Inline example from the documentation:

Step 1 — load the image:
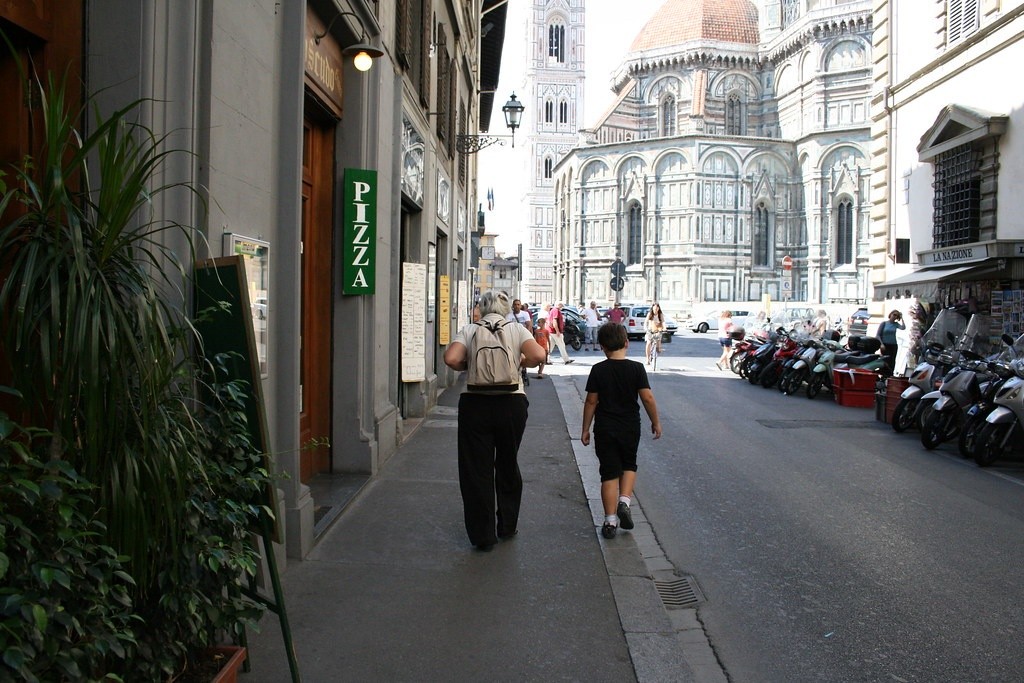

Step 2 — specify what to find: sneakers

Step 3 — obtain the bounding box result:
[602,521,618,539]
[616,502,634,530]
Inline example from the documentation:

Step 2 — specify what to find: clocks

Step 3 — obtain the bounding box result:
[481,246,495,260]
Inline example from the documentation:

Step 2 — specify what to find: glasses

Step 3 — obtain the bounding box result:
[892,313,899,318]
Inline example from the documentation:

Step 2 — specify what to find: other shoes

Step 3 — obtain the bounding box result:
[545,362,553,365]
[716,361,723,371]
[593,348,599,351]
[477,545,493,551]
[499,534,516,541]
[565,360,574,364]
[538,370,543,378]
[585,348,589,351]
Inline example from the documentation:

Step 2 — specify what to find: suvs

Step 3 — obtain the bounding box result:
[849,306,868,336]
[602,306,650,341]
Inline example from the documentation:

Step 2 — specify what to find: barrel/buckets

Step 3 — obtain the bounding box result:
[885,373,912,424]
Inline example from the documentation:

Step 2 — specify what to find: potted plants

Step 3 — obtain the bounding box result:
[0,27,333,683]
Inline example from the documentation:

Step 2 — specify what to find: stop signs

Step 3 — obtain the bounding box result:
[782,257,792,270]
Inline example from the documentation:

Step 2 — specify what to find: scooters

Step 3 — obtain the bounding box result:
[891,330,1024,469]
[727,316,890,399]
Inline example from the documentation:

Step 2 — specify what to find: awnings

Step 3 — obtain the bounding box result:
[874,260,999,302]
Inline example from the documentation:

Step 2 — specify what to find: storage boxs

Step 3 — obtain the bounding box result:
[875,393,887,423]
[831,386,874,408]
[833,368,878,391]
[886,377,911,424]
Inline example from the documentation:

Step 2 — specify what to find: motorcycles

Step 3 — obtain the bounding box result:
[561,312,582,351]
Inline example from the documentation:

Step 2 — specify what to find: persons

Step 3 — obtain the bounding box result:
[581,322,661,539]
[604,302,626,324]
[506,299,551,378]
[642,303,667,365]
[875,310,906,382]
[546,300,575,365]
[473,301,481,323]
[443,290,547,553]
[578,300,601,352]
[716,310,735,371]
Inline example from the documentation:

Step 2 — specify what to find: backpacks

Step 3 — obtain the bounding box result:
[467,320,521,392]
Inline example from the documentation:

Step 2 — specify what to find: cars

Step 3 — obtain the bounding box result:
[685,311,757,333]
[664,317,677,336]
[527,305,609,332]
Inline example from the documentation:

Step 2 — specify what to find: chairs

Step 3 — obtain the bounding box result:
[637,312,644,317]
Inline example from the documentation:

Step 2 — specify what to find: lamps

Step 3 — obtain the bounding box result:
[457,91,525,155]
[314,12,384,72]
